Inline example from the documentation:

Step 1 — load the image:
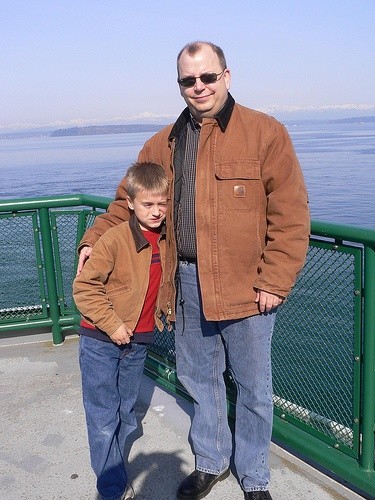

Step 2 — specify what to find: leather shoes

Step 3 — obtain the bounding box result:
[244,492,272,500]
[177,465,230,500]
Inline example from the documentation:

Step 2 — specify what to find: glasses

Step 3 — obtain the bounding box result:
[177,71,225,87]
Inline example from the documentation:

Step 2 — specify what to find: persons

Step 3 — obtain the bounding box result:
[72,39,311,499]
[71,160,180,500]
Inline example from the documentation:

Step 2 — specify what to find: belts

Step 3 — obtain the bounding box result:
[178,255,197,265]
